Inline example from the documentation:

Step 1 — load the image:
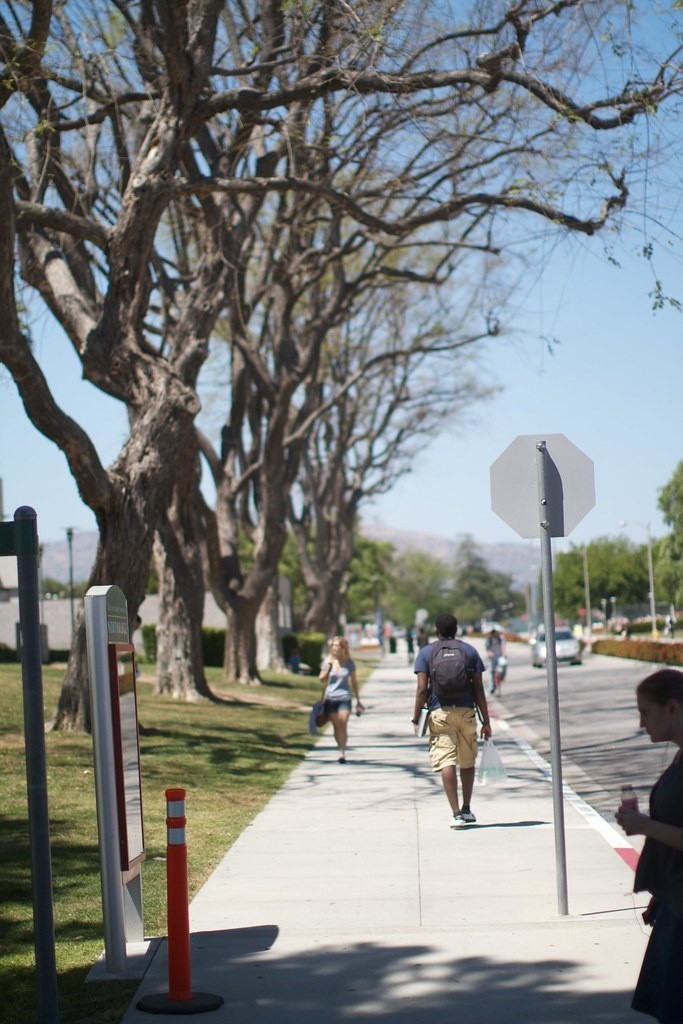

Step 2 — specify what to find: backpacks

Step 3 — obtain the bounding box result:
[431,640,469,699]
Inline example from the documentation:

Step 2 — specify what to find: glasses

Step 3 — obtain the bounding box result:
[331,643,342,647]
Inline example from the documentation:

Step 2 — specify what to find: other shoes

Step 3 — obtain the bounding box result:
[339,757,345,763]
[461,812,476,824]
[451,816,468,830]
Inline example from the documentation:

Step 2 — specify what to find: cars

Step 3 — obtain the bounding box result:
[530,624,581,669]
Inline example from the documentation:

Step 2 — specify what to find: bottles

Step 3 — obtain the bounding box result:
[621,783,641,835]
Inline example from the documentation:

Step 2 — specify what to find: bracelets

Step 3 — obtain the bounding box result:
[411,719,418,725]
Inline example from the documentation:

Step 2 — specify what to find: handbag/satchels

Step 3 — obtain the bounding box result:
[478,736,506,784]
[317,704,328,726]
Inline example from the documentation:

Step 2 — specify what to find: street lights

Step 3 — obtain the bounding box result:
[618,518,657,638]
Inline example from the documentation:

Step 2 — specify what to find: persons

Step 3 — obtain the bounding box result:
[417,627,428,652]
[615,669,683,1024]
[414,613,492,829]
[405,628,415,663]
[486,629,506,694]
[319,636,366,764]
[665,612,677,640]
